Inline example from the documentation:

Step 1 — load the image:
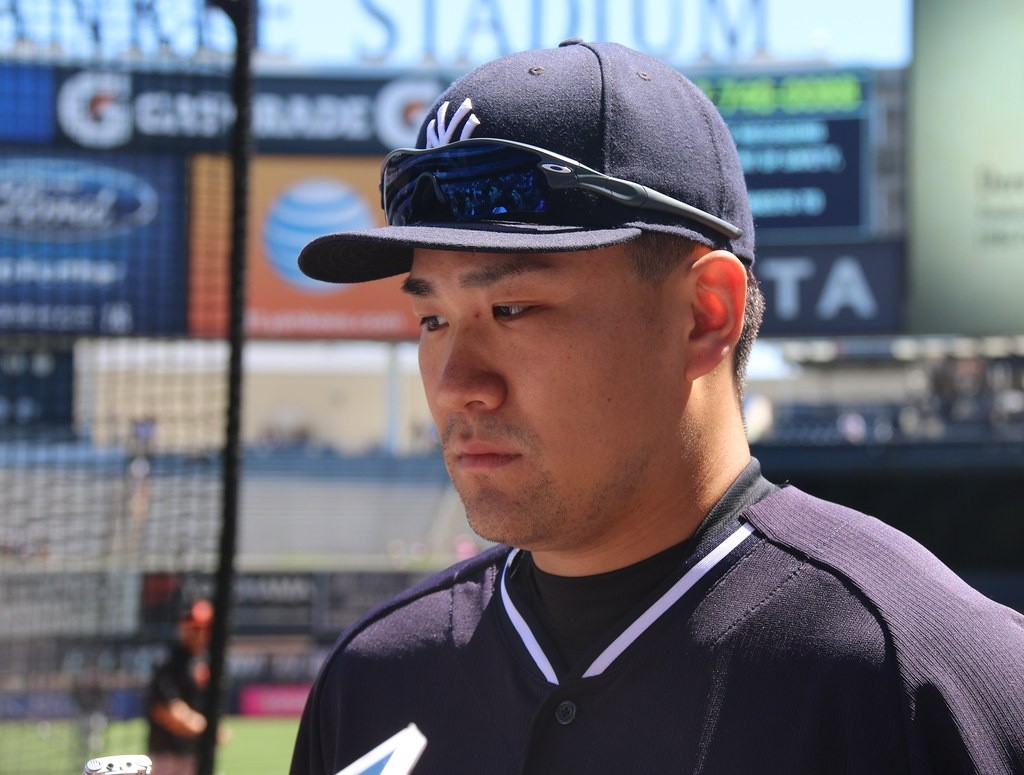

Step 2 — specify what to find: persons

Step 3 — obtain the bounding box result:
[289,36,1023,775]
[142,601,226,774]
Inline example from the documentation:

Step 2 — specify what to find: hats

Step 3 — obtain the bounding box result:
[297,39,756,284]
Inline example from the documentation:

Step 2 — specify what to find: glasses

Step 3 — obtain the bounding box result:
[380,137,744,239]
